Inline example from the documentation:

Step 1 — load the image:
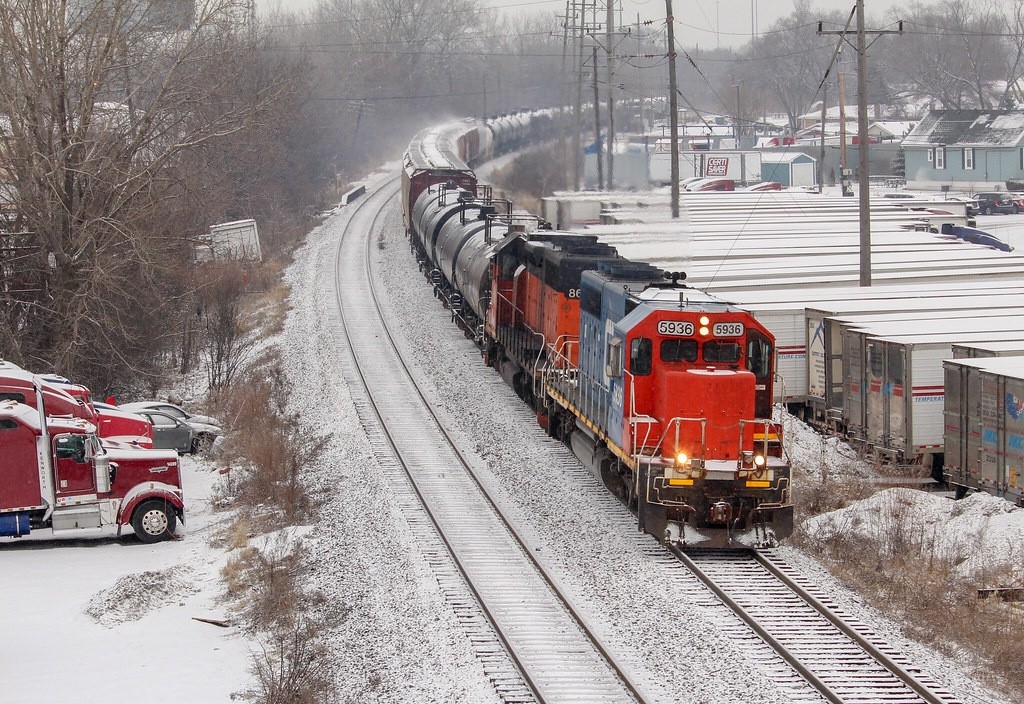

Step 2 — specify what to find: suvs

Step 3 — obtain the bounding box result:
[1009,192,1024,214]
[972,192,1013,215]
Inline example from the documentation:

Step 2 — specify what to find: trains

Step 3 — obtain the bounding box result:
[399,91,797,553]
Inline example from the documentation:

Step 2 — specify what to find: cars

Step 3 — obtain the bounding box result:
[946,197,980,218]
[125,409,223,455]
[754,122,783,133]
[118,401,221,428]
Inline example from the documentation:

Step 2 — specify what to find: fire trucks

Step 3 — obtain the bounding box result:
[0,358,154,450]
[0,398,185,546]
